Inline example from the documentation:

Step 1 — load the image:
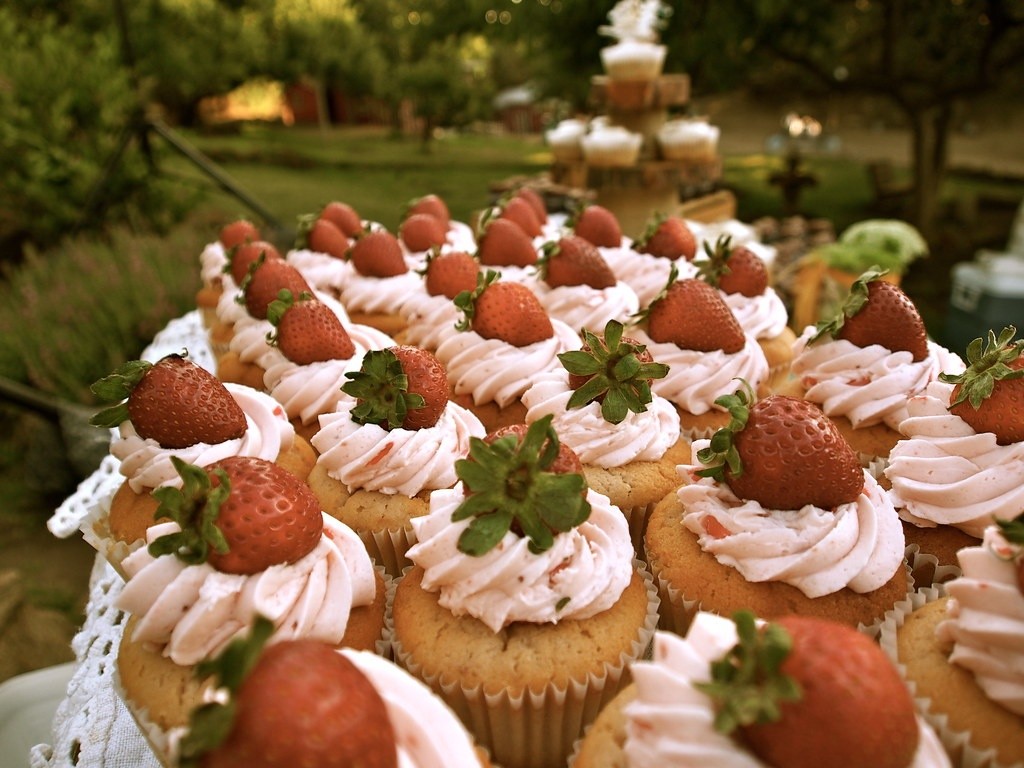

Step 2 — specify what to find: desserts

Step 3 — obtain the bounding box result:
[77,36,1024,768]
